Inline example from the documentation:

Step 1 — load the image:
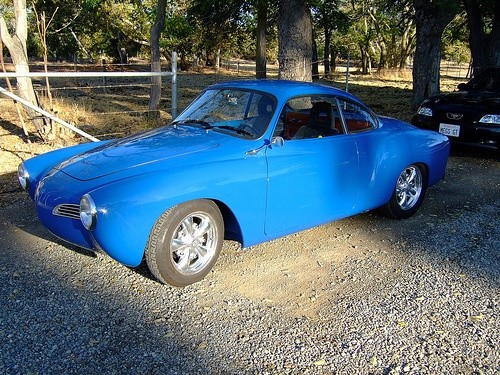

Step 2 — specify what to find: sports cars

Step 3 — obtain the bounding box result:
[17,74,452,288]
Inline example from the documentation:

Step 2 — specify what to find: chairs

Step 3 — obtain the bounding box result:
[237,95,284,139]
[290,101,339,140]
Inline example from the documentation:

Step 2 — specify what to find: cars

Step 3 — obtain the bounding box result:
[410,66,500,162]
